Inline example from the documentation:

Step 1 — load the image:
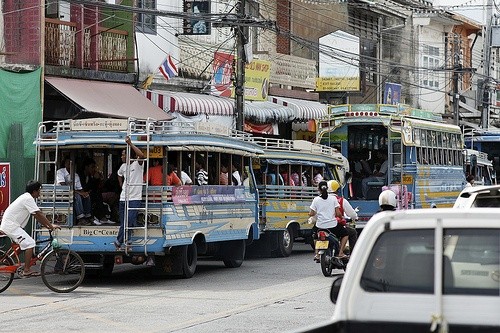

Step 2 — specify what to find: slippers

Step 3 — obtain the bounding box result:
[314,255,320,259]
[23,272,41,276]
[337,255,347,258]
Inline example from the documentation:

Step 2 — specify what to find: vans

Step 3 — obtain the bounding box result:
[441,184,500,288]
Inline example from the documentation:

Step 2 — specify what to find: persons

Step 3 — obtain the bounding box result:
[466,175,474,187]
[267,166,323,199]
[143,154,250,186]
[308,180,358,260]
[0,180,61,277]
[350,149,388,199]
[76,158,116,225]
[115,136,144,249]
[55,157,102,226]
[379,190,397,211]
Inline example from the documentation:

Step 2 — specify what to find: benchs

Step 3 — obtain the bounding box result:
[368,171,388,185]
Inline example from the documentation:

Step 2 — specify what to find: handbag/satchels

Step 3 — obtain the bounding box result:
[308,216,316,225]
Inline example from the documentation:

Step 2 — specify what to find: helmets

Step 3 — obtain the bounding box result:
[327,179,340,193]
[379,189,397,207]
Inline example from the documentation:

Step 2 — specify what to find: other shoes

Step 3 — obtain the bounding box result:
[77,218,96,226]
[100,219,117,224]
[128,243,132,245]
[93,219,102,226]
[115,241,121,249]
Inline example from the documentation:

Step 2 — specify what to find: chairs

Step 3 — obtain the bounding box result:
[403,253,454,289]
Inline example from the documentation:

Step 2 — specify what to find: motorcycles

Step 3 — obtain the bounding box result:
[314,206,360,277]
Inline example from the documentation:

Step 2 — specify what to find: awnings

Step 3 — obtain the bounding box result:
[267,96,328,121]
[43,76,176,132]
[138,88,236,115]
[459,120,498,129]
[220,96,294,122]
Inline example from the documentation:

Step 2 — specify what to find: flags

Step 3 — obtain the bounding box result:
[158,55,178,81]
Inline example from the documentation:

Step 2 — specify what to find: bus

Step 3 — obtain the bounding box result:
[30,116,266,279]
[232,137,353,257]
[314,102,498,232]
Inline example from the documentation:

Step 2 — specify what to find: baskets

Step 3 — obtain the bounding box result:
[53,230,74,244]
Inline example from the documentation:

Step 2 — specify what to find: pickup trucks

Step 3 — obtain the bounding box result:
[293,206,500,333]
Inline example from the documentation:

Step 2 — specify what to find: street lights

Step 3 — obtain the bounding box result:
[377,24,407,104]
[451,78,484,125]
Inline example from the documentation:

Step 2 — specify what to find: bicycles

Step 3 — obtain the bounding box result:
[0,226,86,294]
